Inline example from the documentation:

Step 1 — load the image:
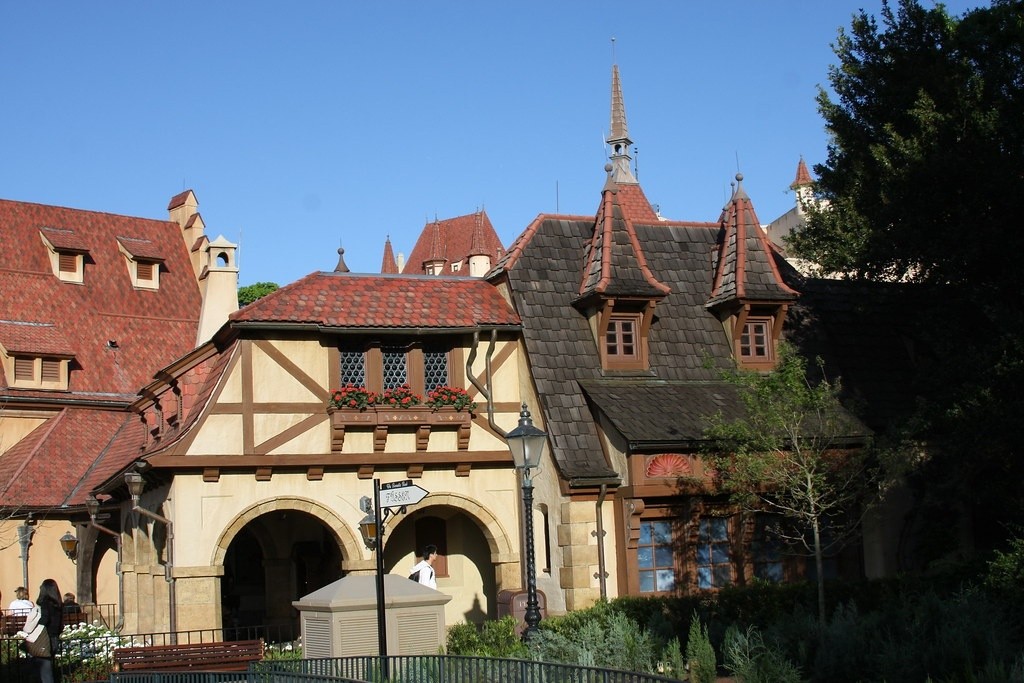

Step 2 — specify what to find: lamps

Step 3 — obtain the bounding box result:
[85,494,99,519]
[124,473,148,507]
[59,529,79,565]
[358,497,386,550]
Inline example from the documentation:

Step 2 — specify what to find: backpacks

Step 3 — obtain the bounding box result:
[408,566,433,583]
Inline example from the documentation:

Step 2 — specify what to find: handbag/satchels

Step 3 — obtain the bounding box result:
[19,625,53,658]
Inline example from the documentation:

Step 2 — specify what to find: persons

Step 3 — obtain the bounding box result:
[22,579,64,683]
[9,586,35,616]
[61,592,81,613]
[407,545,437,591]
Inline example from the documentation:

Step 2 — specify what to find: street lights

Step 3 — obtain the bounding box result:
[503,403,550,650]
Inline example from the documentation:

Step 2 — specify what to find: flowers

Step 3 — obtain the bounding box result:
[327,383,478,411]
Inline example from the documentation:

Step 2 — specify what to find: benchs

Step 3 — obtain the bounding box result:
[116,639,265,672]
[1,613,89,634]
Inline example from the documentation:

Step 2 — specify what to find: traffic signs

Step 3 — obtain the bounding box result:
[379,480,430,508]
